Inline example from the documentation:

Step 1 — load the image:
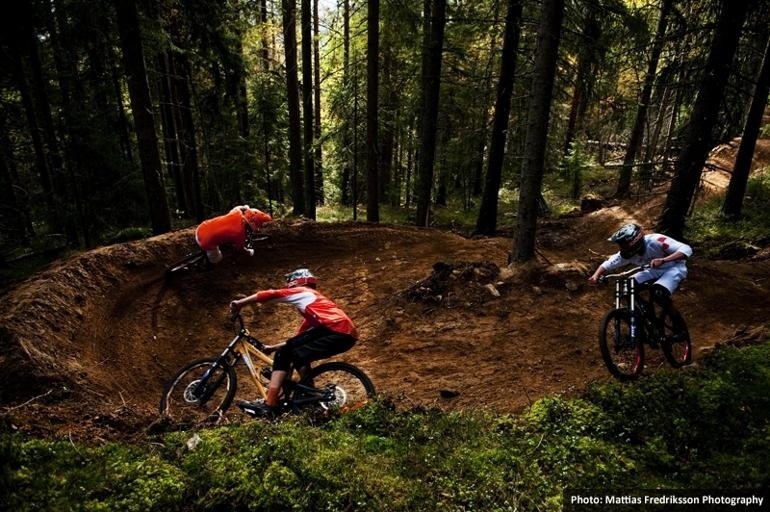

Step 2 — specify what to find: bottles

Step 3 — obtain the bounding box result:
[259,367,273,380]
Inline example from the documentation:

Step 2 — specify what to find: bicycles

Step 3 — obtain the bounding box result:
[164,227,275,277]
[587,257,696,380]
[158,302,379,429]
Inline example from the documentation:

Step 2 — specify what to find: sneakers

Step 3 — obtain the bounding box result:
[672,331,688,342]
[239,399,277,420]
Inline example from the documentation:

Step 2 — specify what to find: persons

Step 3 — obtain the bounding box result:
[195,204,272,264]
[587,223,693,349]
[229,268,358,421]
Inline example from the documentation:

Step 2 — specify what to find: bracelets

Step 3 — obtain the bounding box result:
[660,258,666,264]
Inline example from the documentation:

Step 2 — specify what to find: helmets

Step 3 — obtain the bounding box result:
[245,208,271,230]
[612,224,645,259]
[284,268,319,288]
